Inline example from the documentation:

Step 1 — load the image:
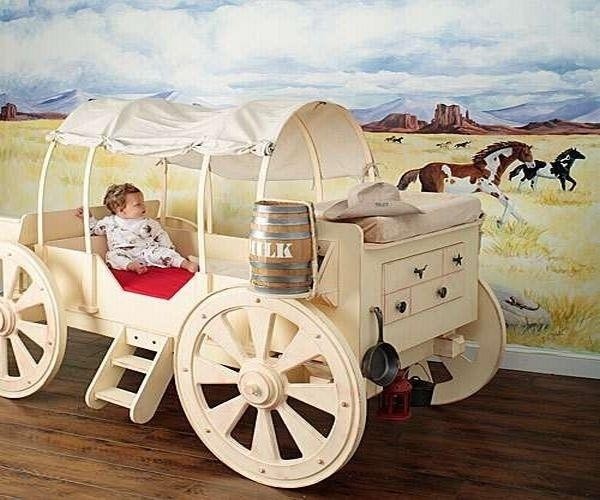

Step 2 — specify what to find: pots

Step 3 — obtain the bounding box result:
[362,306,400,386]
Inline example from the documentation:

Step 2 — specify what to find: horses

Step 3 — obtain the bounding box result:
[383,135,395,143]
[507,146,588,195]
[436,141,451,150]
[454,141,469,149]
[392,136,404,144]
[396,141,537,228]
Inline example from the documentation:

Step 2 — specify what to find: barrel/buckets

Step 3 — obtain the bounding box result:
[403,362,434,408]
[249,200,316,294]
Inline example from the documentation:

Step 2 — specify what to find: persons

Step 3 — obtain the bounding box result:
[74,184,201,278]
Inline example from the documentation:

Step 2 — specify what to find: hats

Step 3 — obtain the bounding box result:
[322,178,425,222]
